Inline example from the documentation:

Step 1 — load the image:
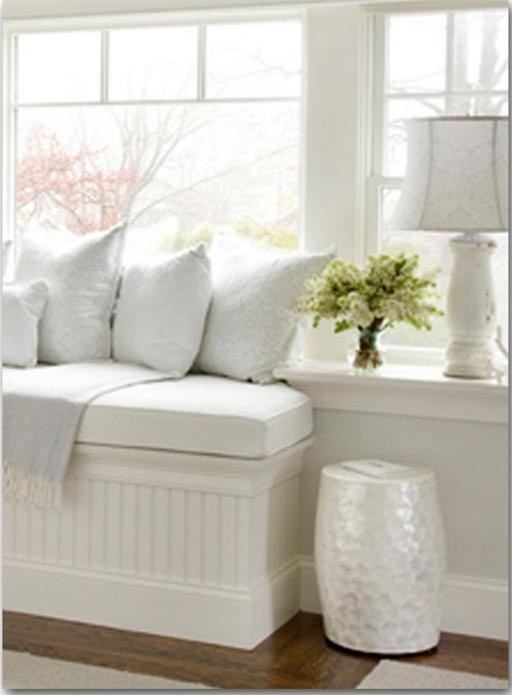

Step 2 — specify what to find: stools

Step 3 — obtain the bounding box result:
[314,459,446,655]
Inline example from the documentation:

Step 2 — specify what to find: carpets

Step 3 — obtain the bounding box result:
[2,650,222,689]
[354,658,508,689]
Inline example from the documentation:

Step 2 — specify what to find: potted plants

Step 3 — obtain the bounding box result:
[293,249,447,370]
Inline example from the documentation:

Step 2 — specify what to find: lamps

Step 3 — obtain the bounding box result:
[390,115,508,378]
